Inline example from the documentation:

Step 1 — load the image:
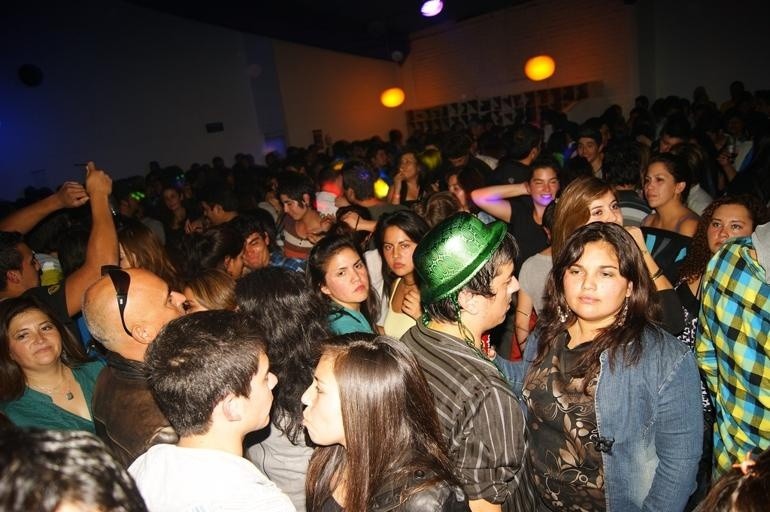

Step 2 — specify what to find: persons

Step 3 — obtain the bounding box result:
[384,111,575,155]
[400,210,532,512]
[603,148,652,227]
[127,310,295,511]
[428,135,494,192]
[230,214,310,272]
[418,148,443,193]
[115,151,269,189]
[576,127,618,181]
[335,159,410,234]
[444,164,483,213]
[333,204,378,253]
[693,444,770,511]
[274,173,331,262]
[693,218,769,480]
[187,185,247,259]
[514,191,563,357]
[119,182,165,244]
[266,136,390,194]
[577,81,770,189]
[378,210,434,344]
[236,268,334,511]
[0,300,108,435]
[313,165,345,217]
[115,223,186,291]
[1,161,118,354]
[305,235,379,336]
[670,142,712,215]
[82,267,187,469]
[296,330,472,512]
[366,144,388,169]
[631,158,701,288]
[674,197,760,506]
[0,421,146,511]
[493,123,546,186]
[184,268,237,313]
[551,176,685,342]
[480,221,704,512]
[387,150,431,213]
[155,184,189,231]
[470,166,561,258]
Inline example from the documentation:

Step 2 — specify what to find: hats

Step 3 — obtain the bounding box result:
[414,212,508,304]
[575,129,600,141]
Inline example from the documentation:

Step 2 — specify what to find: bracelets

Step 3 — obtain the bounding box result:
[649,267,665,282]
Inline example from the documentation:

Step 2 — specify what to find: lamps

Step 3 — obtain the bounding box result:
[417,0,445,19]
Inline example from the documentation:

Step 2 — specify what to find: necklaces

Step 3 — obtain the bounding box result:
[26,370,74,402]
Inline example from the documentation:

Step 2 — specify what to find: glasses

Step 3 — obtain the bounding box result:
[101,265,134,336]
